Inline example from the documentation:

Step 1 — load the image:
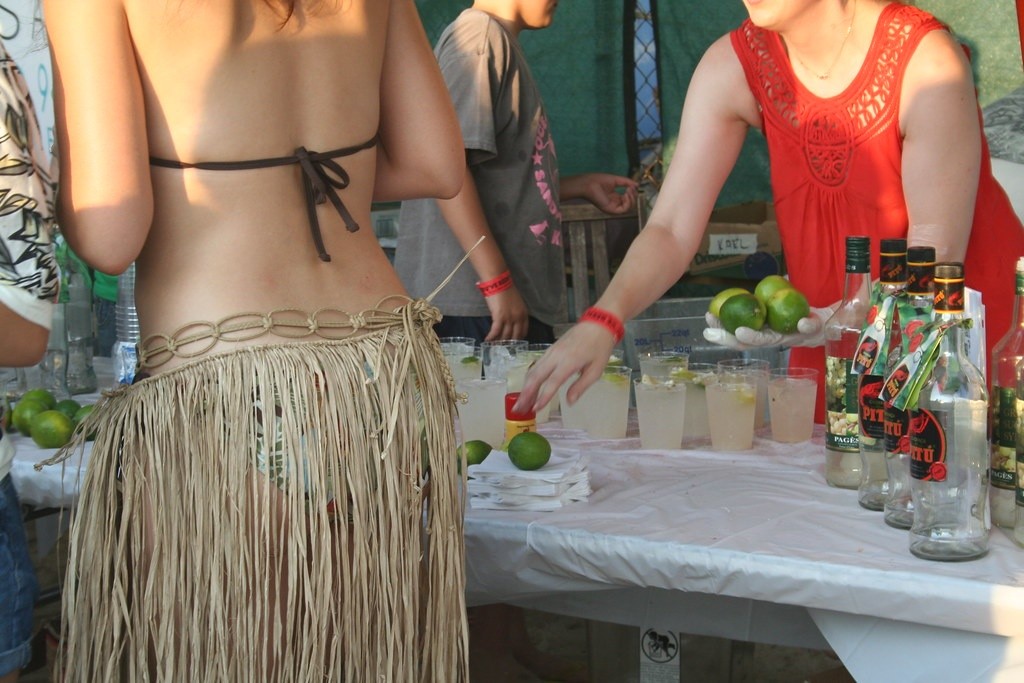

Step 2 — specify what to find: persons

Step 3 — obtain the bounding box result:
[0,0,466,683]
[512,0,1024,424]
[394,0,641,379]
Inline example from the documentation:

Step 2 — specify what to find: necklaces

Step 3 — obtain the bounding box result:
[795,0,857,79]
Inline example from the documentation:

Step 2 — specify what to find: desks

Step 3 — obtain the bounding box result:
[0,355,1024,683]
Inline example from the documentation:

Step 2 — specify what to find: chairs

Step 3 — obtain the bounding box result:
[552,193,657,339]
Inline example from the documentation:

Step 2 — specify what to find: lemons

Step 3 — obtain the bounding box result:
[5,386,100,449]
[507,432,552,470]
[456,440,494,475]
[708,275,811,336]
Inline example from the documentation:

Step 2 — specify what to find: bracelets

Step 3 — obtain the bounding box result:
[475,271,512,297]
[577,307,625,342]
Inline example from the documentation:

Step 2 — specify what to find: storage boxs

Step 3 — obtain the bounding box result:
[686,200,782,277]
[621,297,739,372]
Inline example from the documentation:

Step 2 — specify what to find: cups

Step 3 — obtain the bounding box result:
[441,336,817,449]
[114,255,140,390]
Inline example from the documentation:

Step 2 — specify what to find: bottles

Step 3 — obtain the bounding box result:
[0,225,98,395]
[824,236,1024,563]
